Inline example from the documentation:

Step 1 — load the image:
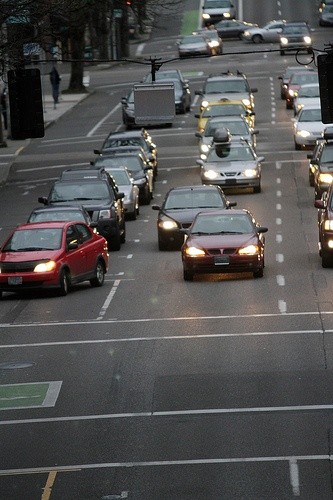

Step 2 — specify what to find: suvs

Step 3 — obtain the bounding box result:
[38,166,125,251]
[194,69,258,111]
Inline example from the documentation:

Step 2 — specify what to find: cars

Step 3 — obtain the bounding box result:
[0,220,110,296]
[178,208,268,282]
[139,70,183,83]
[121,90,135,125]
[102,127,158,156]
[195,115,260,156]
[278,66,333,269]
[194,100,255,134]
[175,0,333,60]
[196,140,265,193]
[90,154,153,204]
[156,79,192,113]
[93,146,151,166]
[151,184,238,252]
[26,205,97,233]
[103,165,140,221]
